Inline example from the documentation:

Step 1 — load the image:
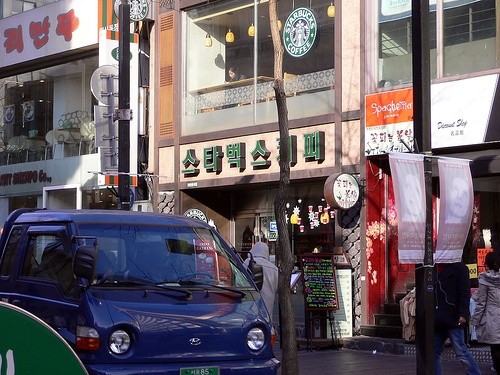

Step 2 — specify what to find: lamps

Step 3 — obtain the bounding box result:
[327,0,335,18]
[204,18,212,47]
[276,0,282,31]
[225,11,235,42]
[248,6,255,36]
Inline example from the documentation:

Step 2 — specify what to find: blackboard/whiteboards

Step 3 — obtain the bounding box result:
[299,253,340,310]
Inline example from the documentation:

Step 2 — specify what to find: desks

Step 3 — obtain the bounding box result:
[196,74,274,113]
[58,127,86,155]
[24,135,45,159]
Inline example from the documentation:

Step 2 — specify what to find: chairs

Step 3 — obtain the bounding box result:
[0,121,96,165]
[33,241,73,282]
[268,72,300,101]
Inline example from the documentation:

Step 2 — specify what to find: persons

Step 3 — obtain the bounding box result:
[471,251,500,375]
[431,262,481,375]
[241,241,279,324]
[222,66,247,110]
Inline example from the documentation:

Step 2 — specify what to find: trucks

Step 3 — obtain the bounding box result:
[0,206,281,375]
